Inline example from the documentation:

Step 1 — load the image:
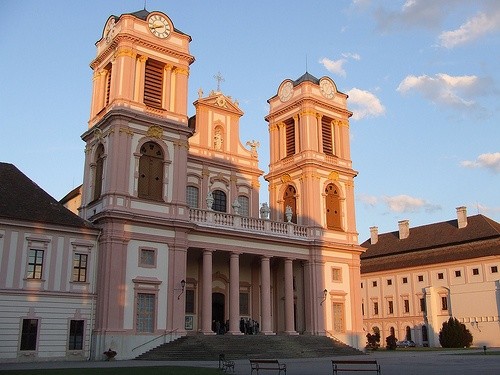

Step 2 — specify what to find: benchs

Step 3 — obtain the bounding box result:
[332,360,381,375]
[250,359,288,375]
[219,353,236,372]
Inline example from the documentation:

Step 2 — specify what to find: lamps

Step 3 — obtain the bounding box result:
[178,280,185,300]
[321,289,328,304]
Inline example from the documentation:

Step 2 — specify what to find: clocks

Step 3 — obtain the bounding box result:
[103,15,117,44]
[146,11,175,39]
[319,76,338,99]
[277,79,294,102]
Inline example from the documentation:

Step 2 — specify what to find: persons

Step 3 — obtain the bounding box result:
[239,317,259,336]
[212,319,229,335]
